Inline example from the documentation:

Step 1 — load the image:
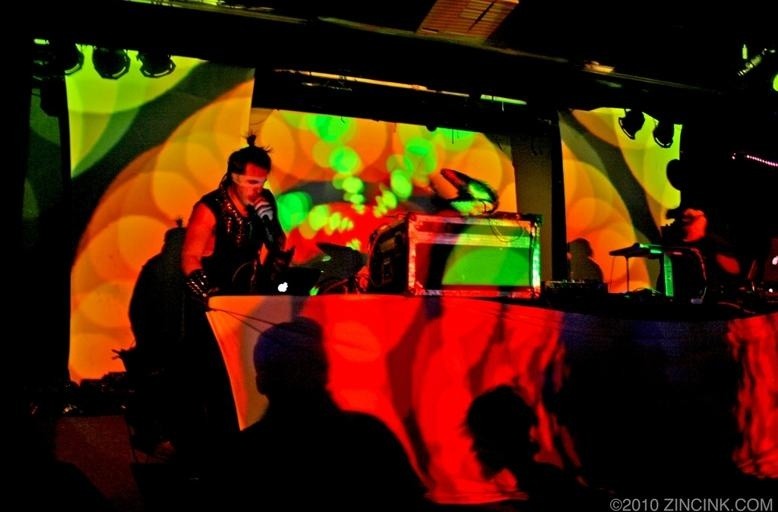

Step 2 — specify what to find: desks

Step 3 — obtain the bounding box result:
[206,291,774,505]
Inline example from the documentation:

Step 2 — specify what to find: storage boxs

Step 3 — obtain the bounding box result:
[370,215,543,298]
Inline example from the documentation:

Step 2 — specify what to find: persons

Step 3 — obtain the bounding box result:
[181,132,285,299]
[656,204,711,305]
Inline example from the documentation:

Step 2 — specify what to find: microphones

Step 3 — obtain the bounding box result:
[251,194,276,243]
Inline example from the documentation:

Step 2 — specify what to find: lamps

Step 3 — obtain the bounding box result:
[43,41,84,77]
[92,50,131,81]
[137,52,177,80]
[620,108,646,141]
[652,120,675,148]
